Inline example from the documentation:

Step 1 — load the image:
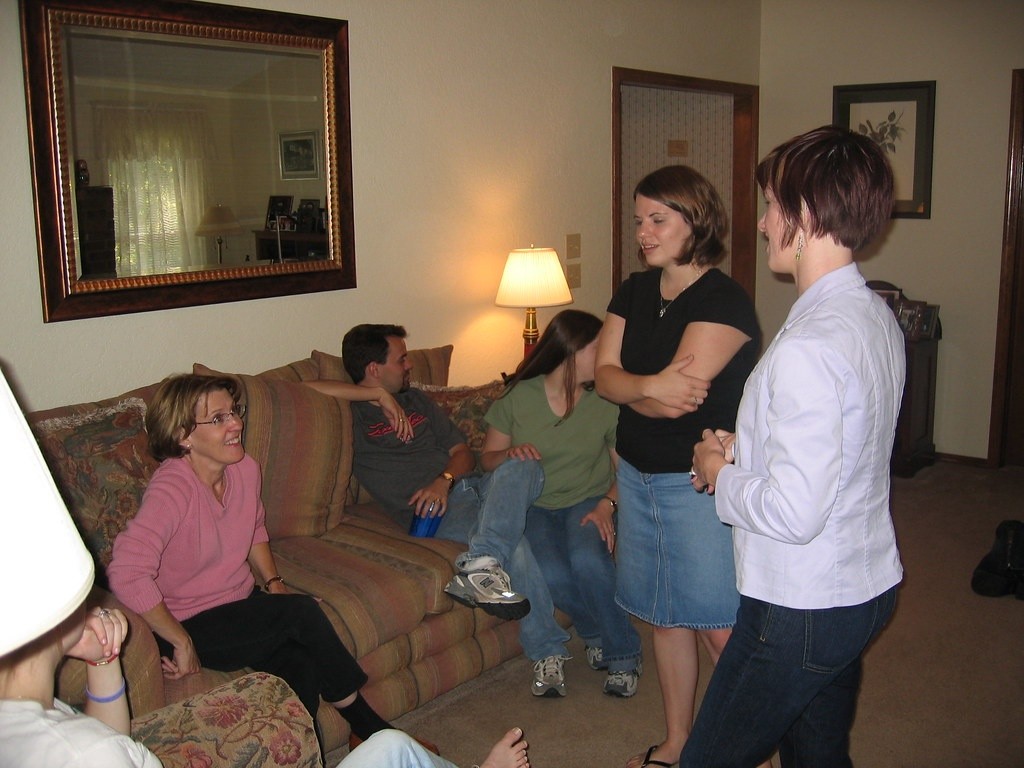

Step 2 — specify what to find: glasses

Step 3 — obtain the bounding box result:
[196,404,247,427]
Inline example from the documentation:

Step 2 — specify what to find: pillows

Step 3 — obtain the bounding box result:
[194,360,345,540]
[409,376,507,469]
[24,397,168,586]
[251,356,319,382]
[311,345,455,387]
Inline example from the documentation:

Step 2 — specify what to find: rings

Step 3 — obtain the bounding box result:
[98,609,111,616]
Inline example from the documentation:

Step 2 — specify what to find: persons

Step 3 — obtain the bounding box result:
[673,122,911,768]
[592,162,766,767]
[0,586,533,768]
[301,324,574,699]
[482,307,646,698]
[106,372,441,765]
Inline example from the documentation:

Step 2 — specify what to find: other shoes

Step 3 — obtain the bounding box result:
[348,732,441,757]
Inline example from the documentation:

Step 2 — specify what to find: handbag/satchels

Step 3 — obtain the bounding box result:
[968,520,1023,600]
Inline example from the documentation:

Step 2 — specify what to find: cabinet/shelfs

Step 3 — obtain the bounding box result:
[252,230,329,263]
[75,186,117,279]
[887,336,936,480]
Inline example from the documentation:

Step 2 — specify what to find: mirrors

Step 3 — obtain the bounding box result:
[18,0,357,325]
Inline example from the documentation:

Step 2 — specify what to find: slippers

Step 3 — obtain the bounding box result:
[624,745,680,768]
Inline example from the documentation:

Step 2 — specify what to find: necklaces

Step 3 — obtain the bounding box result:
[657,262,707,318]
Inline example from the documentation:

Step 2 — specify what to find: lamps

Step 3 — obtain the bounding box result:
[195,202,246,266]
[495,243,575,361]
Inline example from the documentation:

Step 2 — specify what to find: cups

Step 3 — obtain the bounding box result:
[408,496,445,538]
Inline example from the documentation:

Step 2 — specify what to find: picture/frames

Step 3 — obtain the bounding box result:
[299,199,320,233]
[278,129,322,182]
[833,80,940,222]
[265,195,294,232]
[919,305,940,339]
[894,298,927,343]
[278,215,297,232]
[869,286,902,311]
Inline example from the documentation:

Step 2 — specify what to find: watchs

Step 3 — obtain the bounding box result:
[604,494,618,513]
[437,473,455,488]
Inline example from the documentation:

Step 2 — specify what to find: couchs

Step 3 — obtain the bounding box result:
[22,345,574,748]
[128,671,327,768]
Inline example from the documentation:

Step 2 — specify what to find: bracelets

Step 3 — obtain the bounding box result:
[85,679,127,705]
[264,575,283,589]
[87,655,120,669]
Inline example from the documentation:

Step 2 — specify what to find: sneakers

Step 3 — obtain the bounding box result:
[585,647,604,670]
[443,568,531,621]
[530,647,568,697]
[603,662,643,697]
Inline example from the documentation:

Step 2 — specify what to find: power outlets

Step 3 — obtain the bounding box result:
[566,262,581,290]
